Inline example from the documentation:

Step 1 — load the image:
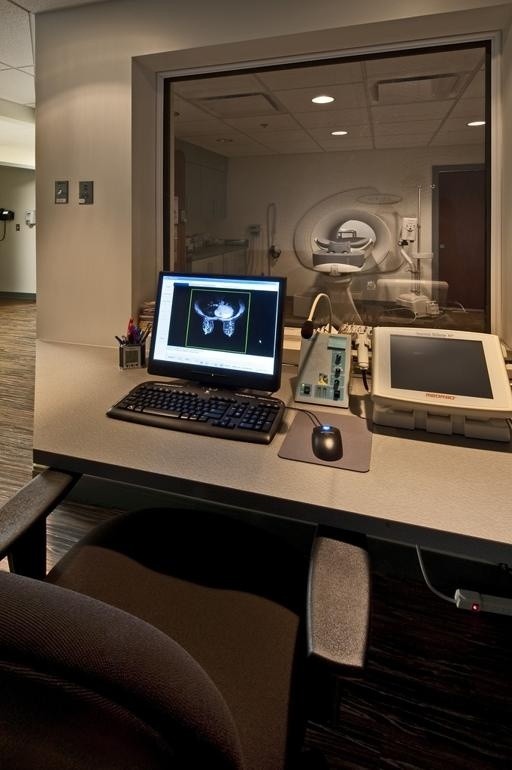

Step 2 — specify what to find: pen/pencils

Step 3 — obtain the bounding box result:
[115,317,152,345]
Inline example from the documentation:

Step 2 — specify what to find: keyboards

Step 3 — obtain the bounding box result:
[106,381,285,444]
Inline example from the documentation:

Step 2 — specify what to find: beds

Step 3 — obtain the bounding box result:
[313,238,372,273]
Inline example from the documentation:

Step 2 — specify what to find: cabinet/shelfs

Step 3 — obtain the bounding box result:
[188,246,248,275]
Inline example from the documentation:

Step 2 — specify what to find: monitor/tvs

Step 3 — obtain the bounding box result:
[370,326,512,419]
[147,271,287,397]
[376,279,449,309]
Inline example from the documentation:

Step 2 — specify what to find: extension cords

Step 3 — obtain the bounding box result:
[454,588,512,616]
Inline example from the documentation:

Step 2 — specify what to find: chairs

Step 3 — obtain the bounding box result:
[0,468,370,770]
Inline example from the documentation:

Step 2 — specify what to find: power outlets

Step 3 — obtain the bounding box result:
[78,181,93,204]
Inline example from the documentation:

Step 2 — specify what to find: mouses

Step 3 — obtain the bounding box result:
[312,426,343,461]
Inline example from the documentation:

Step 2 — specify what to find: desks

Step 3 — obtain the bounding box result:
[32,341,512,566]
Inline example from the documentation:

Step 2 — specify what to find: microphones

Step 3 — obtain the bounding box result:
[294,293,352,410]
